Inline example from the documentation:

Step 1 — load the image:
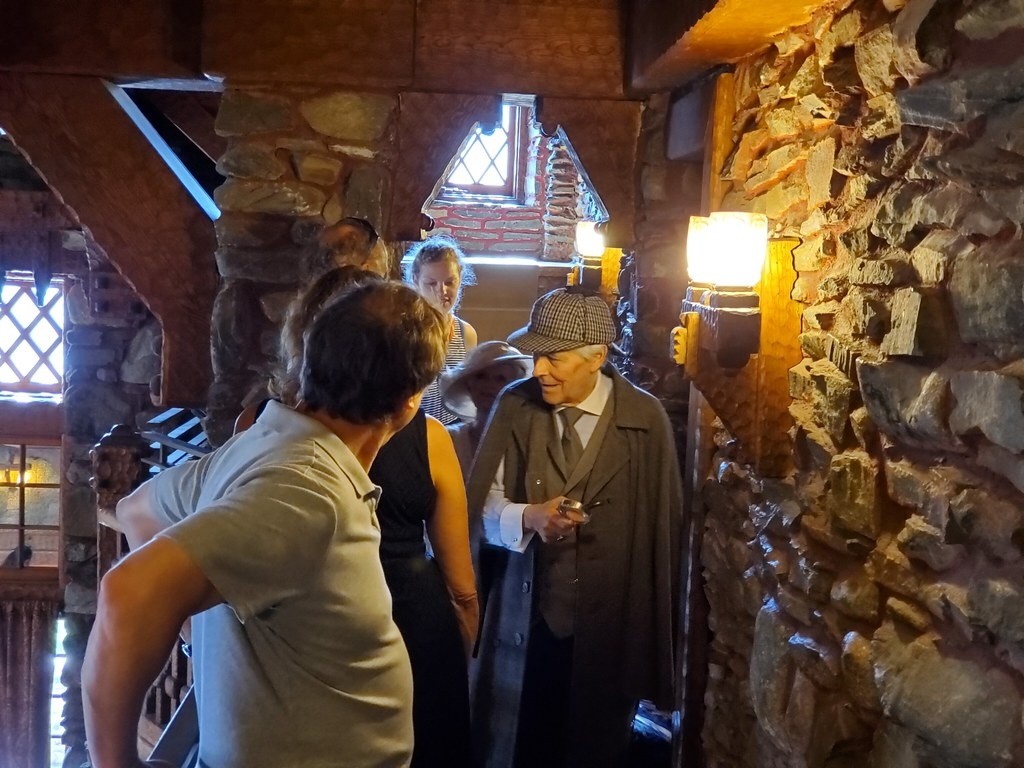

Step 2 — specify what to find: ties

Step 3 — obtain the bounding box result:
[559,407,585,471]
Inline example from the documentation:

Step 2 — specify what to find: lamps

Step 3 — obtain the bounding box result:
[704,212,766,308]
[685,215,709,303]
[574,219,602,288]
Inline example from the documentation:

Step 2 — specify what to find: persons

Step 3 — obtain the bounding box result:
[440,338,536,486]
[402,232,480,427]
[234,265,480,768]
[81,280,453,768]
[456,287,683,768]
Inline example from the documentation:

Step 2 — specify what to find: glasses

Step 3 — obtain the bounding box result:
[348,217,378,265]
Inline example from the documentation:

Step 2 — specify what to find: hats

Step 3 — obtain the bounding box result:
[504,284,617,358]
[443,337,534,419]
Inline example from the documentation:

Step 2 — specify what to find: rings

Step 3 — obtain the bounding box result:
[557,534,565,542]
